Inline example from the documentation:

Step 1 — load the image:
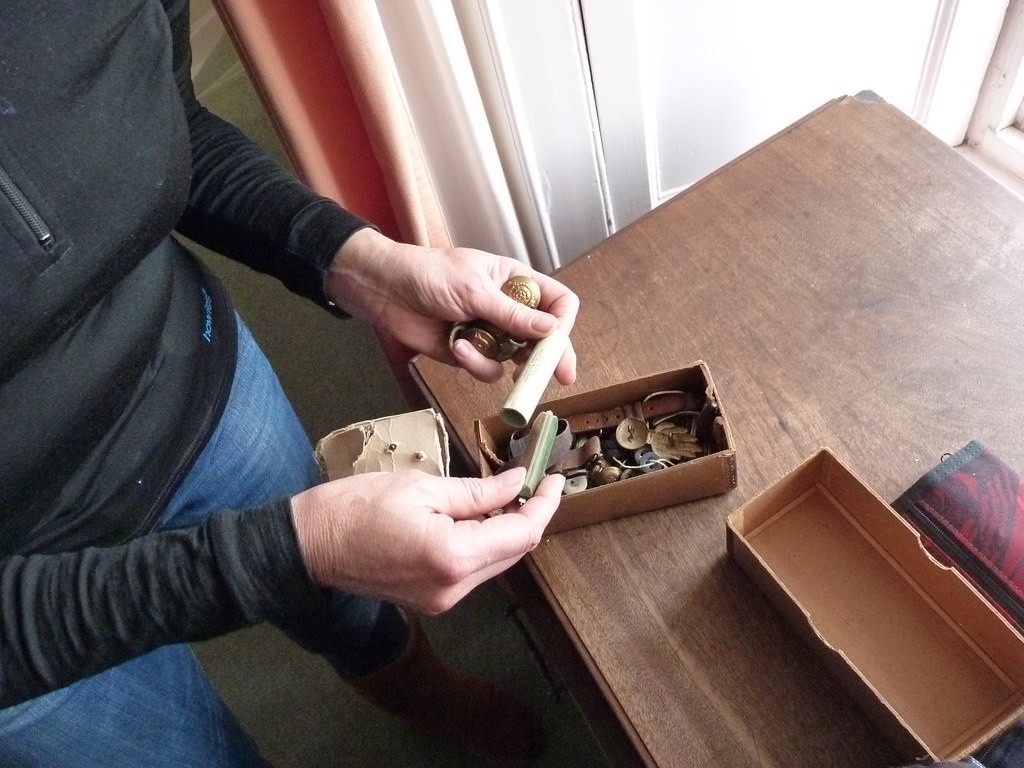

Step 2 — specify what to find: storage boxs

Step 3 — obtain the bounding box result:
[474,359,737,535]
[727,446,1024,765]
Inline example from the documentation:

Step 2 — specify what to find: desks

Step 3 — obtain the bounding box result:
[407,90,1024,768]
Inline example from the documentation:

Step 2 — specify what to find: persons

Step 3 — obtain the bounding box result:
[0,1,584,768]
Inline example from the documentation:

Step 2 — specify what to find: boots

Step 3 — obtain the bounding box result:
[346,604,546,768]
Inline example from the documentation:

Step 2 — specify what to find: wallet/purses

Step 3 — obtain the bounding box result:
[890,438,1024,634]
